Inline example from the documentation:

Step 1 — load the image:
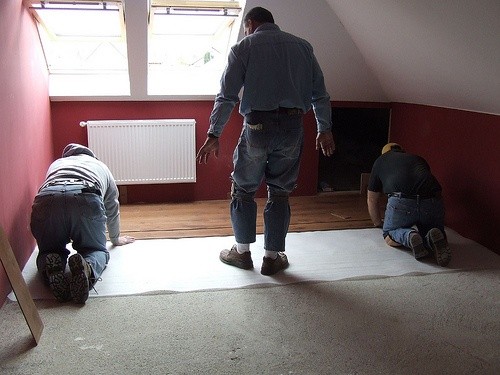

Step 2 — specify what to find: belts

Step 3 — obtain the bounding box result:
[390,193,419,199]
[272,108,303,114]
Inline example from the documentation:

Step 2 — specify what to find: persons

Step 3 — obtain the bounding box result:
[196,6,335,276]
[29,144,135,303]
[367,143,453,266]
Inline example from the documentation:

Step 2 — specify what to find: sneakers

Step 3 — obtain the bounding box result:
[45,252,66,298]
[69,253,91,305]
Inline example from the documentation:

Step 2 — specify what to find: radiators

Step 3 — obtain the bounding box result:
[79,119,197,185]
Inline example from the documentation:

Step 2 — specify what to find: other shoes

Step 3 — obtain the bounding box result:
[220,244,253,269]
[409,232,430,261]
[261,252,288,276]
[429,228,450,267]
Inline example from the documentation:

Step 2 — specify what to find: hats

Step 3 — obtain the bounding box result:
[382,143,403,154]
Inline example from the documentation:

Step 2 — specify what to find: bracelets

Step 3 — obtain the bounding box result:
[207,134,219,138]
